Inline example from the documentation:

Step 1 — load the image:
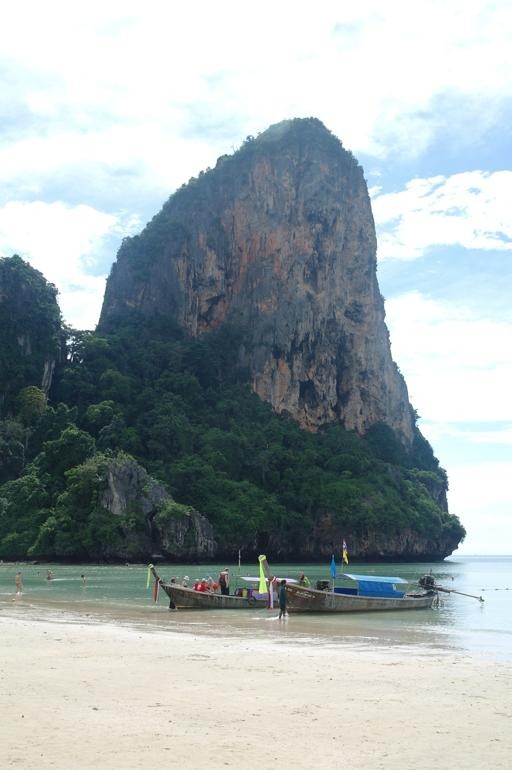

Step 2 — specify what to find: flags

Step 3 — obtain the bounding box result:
[331,557,336,578]
[342,540,349,564]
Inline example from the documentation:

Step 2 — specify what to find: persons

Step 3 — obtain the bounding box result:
[15,572,24,594]
[218,568,230,595]
[298,571,309,589]
[81,574,85,586]
[171,575,218,594]
[278,580,289,620]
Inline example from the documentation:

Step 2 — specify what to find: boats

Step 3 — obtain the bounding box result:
[148,563,299,609]
[259,554,438,614]
